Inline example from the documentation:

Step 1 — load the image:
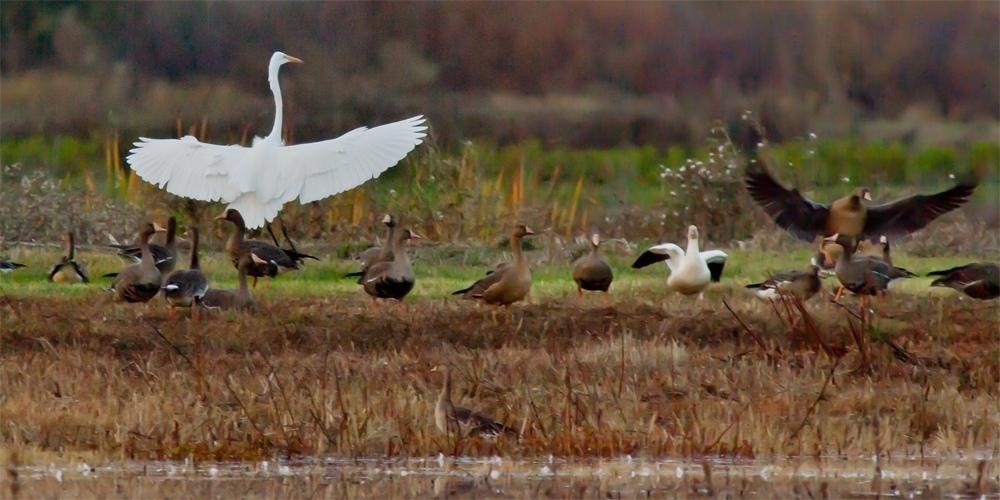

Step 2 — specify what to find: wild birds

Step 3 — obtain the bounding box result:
[927,261,1000,300]
[572,225,613,305]
[819,240,910,305]
[99,208,322,323]
[356,228,423,321]
[864,235,918,277]
[631,224,729,310]
[450,222,536,327]
[743,151,980,276]
[124,51,430,267]
[340,213,397,280]
[0,260,29,273]
[47,229,90,286]
[744,265,828,304]
